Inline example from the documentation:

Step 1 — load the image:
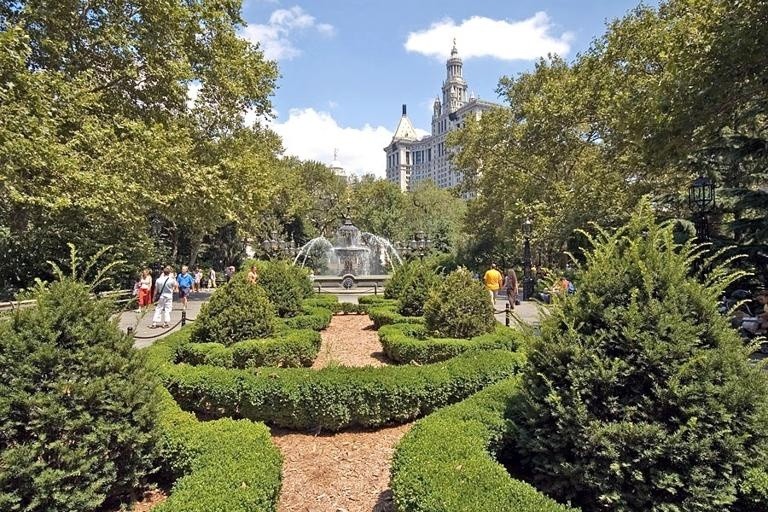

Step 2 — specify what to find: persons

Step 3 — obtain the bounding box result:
[560,276,575,296]
[483,264,503,308]
[133,265,258,328]
[503,269,518,309]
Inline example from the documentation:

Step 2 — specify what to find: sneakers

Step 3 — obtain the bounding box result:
[134,309,168,328]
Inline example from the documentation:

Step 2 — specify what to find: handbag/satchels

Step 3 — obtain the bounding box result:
[515,299,520,305]
[154,293,160,302]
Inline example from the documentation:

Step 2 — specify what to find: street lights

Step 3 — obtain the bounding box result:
[415,230,424,261]
[521,216,534,300]
[688,176,716,282]
